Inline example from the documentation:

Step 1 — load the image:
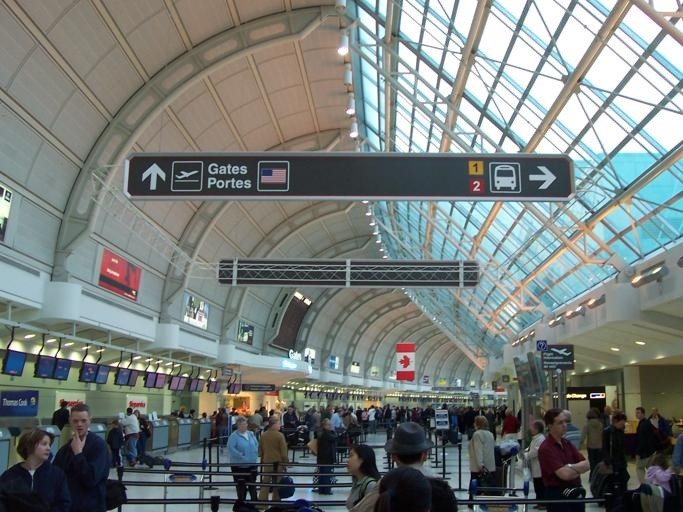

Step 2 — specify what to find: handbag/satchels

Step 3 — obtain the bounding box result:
[522,467,532,482]
[562,484,587,499]
[477,468,498,487]
[142,419,152,437]
[277,477,297,499]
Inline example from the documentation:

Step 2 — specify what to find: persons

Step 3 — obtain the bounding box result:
[52,402,110,511]
[0,429,71,511]
[51,401,69,431]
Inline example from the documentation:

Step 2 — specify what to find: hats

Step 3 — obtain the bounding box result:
[384,421,435,456]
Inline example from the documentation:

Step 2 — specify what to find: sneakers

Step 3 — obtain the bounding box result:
[319,491,334,495]
[533,505,547,510]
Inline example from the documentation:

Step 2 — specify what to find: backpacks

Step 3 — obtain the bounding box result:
[650,424,671,452]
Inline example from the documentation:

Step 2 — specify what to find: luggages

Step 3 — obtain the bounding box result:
[589,461,625,497]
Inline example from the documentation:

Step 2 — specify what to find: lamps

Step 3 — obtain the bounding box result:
[509,255,682,350]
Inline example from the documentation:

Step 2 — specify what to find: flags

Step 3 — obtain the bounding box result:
[395,343,414,381]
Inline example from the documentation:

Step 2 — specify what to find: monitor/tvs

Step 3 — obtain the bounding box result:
[144,373,241,394]
[304,391,382,401]
[53,358,72,380]
[95,365,111,385]
[114,368,131,386]
[78,362,97,383]
[127,370,139,387]
[1,350,28,377]
[33,355,55,378]
